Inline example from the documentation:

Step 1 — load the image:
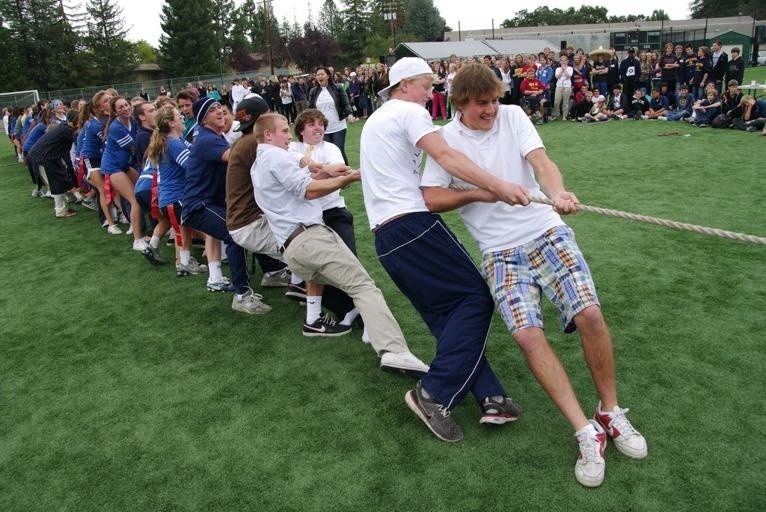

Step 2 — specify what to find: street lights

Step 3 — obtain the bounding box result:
[366,55,372,67]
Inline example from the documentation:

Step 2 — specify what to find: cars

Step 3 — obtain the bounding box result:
[750,52,766,67]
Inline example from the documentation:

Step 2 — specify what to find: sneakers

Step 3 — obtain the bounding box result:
[480,396,521,425]
[575,400,648,487]
[380,352,464,442]
[611,110,668,121]
[33,190,133,237]
[205,269,352,337]
[131,235,229,276]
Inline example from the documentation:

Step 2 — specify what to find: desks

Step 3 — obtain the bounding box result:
[736,84,766,99]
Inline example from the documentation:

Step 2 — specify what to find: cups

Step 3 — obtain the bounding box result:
[750,80,758,87]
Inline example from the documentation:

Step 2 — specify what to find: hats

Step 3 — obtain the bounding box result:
[378,57,433,97]
[233,93,269,132]
[629,48,634,52]
[192,97,221,126]
[350,72,357,77]
